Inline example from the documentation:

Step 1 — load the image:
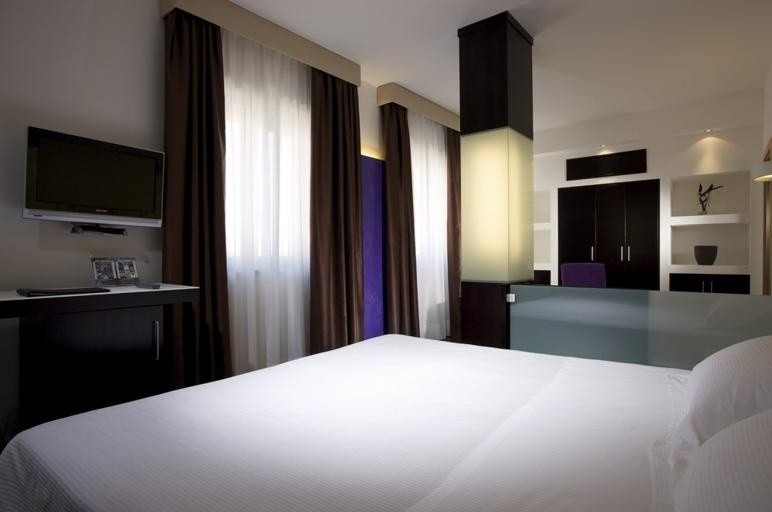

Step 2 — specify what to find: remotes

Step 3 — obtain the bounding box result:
[136,282,160,289]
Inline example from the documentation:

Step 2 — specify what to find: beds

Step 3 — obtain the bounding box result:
[0,334,690,512]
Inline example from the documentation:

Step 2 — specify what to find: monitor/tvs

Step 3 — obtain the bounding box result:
[22,125,166,235]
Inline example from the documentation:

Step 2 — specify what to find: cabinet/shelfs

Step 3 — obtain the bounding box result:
[669,273,750,294]
[595,179,660,290]
[670,213,750,274]
[558,185,595,290]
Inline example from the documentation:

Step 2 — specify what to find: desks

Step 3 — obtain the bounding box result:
[0,283,202,319]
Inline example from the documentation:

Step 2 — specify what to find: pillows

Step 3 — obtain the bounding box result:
[652,335,772,439]
[645,409,772,512]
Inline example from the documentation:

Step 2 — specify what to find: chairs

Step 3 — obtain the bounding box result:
[561,263,606,289]
